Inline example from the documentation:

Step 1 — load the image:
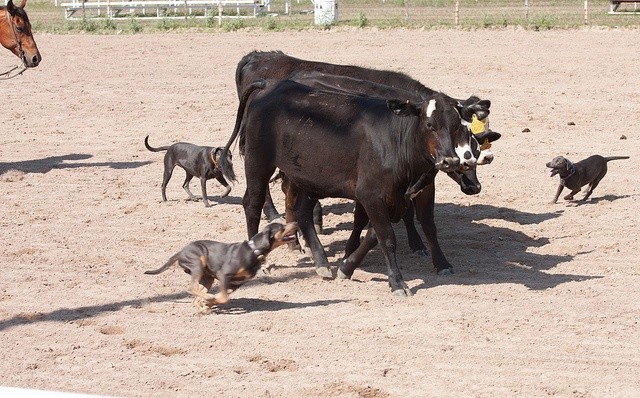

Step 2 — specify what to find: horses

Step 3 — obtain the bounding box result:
[0,0,42,68]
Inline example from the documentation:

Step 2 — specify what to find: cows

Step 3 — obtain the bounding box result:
[282,71,501,276]
[235,51,494,234]
[218,79,490,298]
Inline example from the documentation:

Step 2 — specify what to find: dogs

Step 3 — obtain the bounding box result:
[144,222,298,315]
[545,155,630,205]
[144,135,233,207]
